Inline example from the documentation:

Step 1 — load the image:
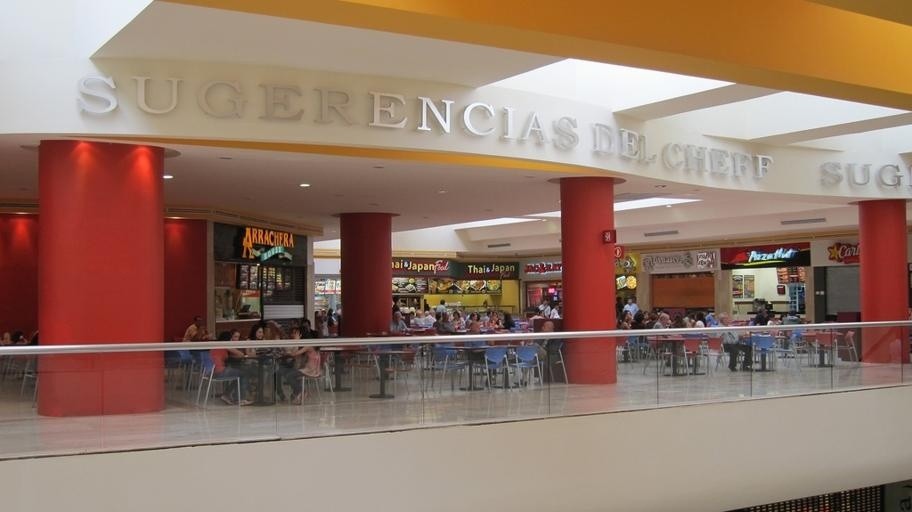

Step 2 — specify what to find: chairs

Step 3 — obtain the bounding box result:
[0,313,859,411]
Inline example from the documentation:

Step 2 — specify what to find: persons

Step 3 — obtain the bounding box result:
[389,293,565,386]
[615,293,812,374]
[24,330,39,374]
[172,306,343,407]
[0,330,29,379]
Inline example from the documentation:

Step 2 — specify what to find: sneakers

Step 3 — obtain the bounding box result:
[291,388,308,405]
[240,400,254,406]
[220,394,234,405]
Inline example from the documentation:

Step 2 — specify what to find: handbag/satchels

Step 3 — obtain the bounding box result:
[285,356,294,367]
